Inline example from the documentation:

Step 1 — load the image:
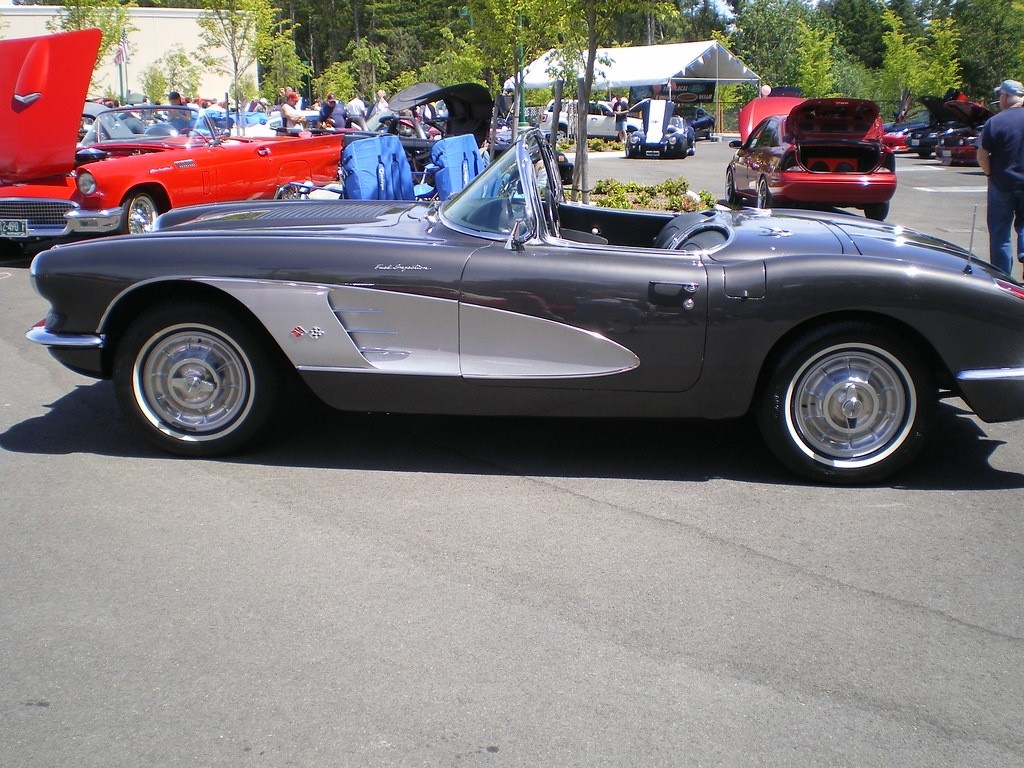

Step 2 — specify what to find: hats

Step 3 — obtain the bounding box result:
[260,98,267,103]
[327,94,335,101]
[994,79,1024,97]
[289,92,299,101]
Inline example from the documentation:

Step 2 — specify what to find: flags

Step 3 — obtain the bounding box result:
[114,28,129,65]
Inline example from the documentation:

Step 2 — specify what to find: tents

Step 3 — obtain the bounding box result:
[504,39,761,137]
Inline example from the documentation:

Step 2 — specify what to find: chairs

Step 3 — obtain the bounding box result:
[289,135,417,199]
[388,115,427,139]
[411,134,484,202]
[345,116,370,132]
[653,214,709,249]
[674,224,729,251]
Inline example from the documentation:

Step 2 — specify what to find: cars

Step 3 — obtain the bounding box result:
[0,28,344,259]
[903,95,947,159]
[726,96,897,221]
[883,87,970,151]
[672,106,716,140]
[934,100,997,167]
[539,98,651,143]
[75,81,574,194]
[625,100,696,160]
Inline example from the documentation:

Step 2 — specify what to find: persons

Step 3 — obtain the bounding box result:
[973,80,1024,284]
[93,87,448,134]
[612,93,630,145]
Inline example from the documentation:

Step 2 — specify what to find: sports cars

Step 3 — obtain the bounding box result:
[25,128,1024,489]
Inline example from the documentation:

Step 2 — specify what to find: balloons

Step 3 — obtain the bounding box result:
[762,85,771,95]
